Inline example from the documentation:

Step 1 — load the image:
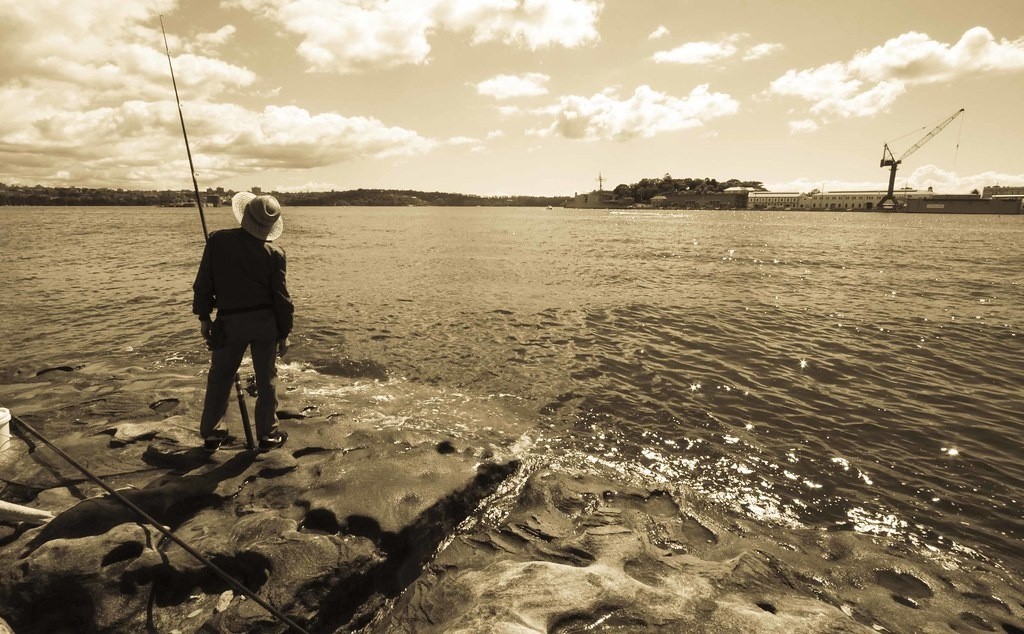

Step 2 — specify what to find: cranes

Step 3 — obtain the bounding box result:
[876,108,964,210]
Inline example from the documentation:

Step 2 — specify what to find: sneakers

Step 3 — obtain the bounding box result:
[258,430,289,452]
[204,422,229,452]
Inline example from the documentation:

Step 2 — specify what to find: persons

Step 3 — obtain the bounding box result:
[192,190,296,453]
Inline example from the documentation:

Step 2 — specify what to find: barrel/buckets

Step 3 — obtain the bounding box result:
[0,407,12,452]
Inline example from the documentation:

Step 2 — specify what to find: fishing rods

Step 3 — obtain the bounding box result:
[0,403,310,634]
[159,12,255,450]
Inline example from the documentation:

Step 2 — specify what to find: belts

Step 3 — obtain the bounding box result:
[220,304,275,315]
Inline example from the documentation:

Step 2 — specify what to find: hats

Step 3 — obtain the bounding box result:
[232,192,283,241]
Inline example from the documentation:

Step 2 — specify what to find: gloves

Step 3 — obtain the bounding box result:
[277,337,290,357]
[201,320,215,339]
